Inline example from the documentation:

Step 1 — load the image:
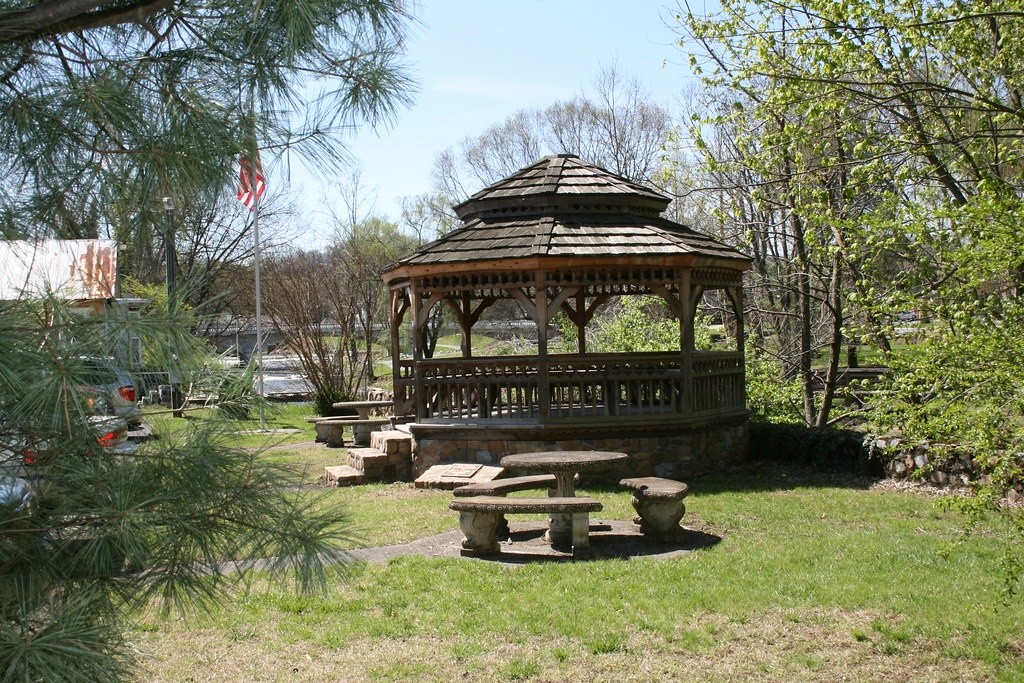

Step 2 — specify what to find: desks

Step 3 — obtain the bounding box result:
[332,401,395,420]
[499,450,630,544]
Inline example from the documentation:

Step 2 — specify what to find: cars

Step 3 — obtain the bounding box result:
[0,416,137,506]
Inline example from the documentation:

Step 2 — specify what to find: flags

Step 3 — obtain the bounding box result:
[237,109,265,213]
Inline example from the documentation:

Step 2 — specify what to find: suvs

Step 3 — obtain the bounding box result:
[29,353,141,428]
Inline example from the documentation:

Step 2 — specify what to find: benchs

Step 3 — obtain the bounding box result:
[453,472,580,541]
[618,476,691,546]
[316,419,391,448]
[305,414,369,442]
[398,373,479,415]
[449,495,605,561]
